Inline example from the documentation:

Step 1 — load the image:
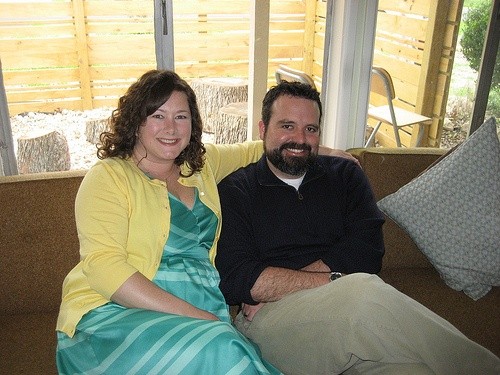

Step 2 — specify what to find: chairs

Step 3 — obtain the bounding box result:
[275,64,433,148]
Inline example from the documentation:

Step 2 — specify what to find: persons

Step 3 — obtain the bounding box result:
[217,80,500,375]
[56,69,363,375]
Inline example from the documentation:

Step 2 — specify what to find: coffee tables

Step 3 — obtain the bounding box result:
[189,77,248,134]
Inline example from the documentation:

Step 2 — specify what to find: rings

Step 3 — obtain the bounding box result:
[243,313,248,317]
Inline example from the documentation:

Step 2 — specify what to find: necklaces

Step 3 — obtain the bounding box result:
[163,166,175,182]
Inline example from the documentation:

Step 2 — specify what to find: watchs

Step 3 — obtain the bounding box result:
[328,271,343,282]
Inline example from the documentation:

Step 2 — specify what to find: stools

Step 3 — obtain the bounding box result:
[216,102,248,144]
[85,117,112,144]
[16,128,70,175]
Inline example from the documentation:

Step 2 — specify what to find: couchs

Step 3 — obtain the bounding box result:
[0,147,500,375]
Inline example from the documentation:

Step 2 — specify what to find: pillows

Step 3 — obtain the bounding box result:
[376,118,500,302]
